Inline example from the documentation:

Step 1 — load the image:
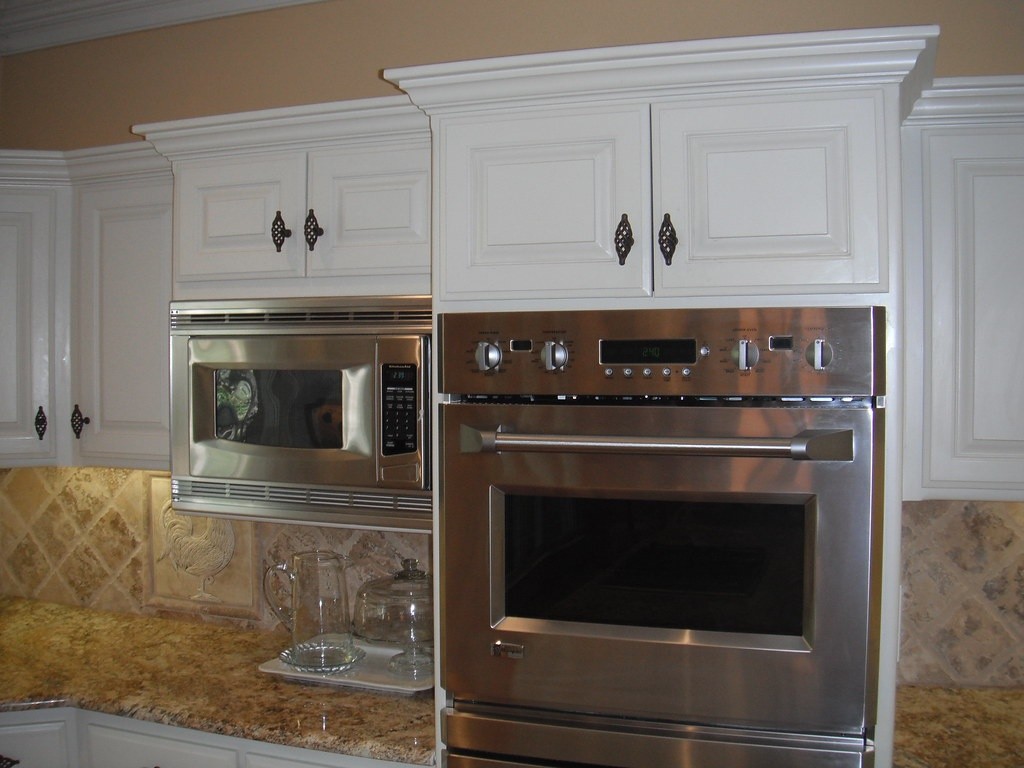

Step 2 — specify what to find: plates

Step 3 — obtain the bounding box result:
[259,632,435,691]
[279,644,366,673]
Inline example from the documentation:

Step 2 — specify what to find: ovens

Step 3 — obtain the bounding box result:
[439,308,885,768]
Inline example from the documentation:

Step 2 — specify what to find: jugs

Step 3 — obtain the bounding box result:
[263,551,351,664]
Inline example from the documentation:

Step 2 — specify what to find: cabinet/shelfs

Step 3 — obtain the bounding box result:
[71,163,170,472]
[172,131,432,300]
[900,124,1024,501]
[1,720,71,767]
[0,177,70,471]
[431,78,898,313]
[88,721,332,767]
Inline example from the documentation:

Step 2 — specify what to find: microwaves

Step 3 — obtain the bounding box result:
[169,297,434,528]
